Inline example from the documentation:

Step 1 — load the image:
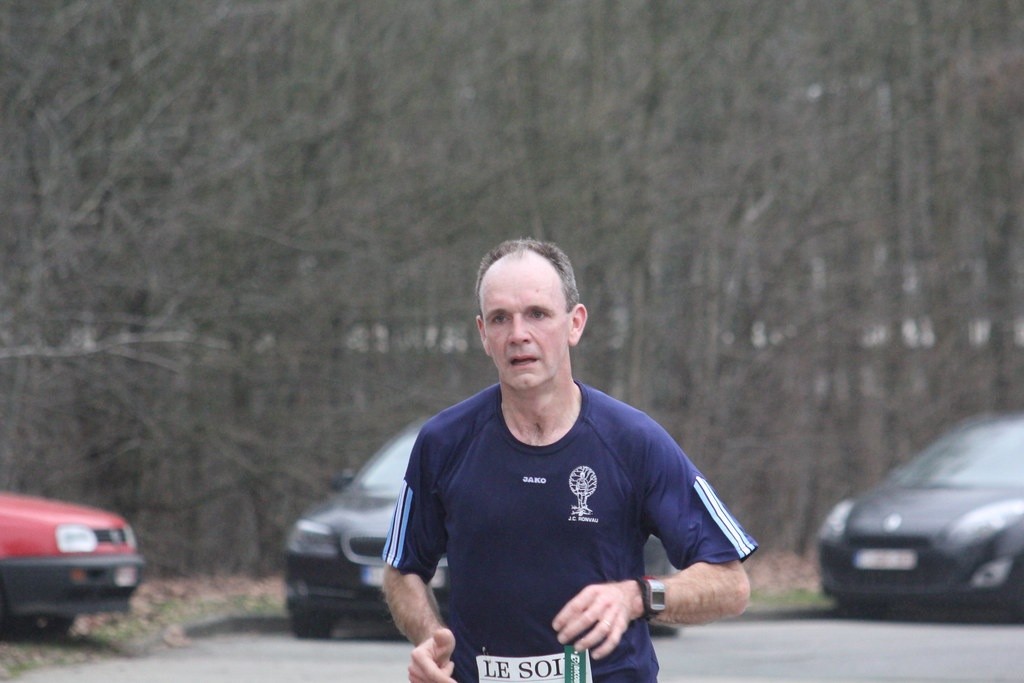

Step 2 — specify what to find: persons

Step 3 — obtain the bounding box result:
[381,238,759,683]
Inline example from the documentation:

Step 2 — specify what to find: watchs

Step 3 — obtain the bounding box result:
[637,576,665,621]
